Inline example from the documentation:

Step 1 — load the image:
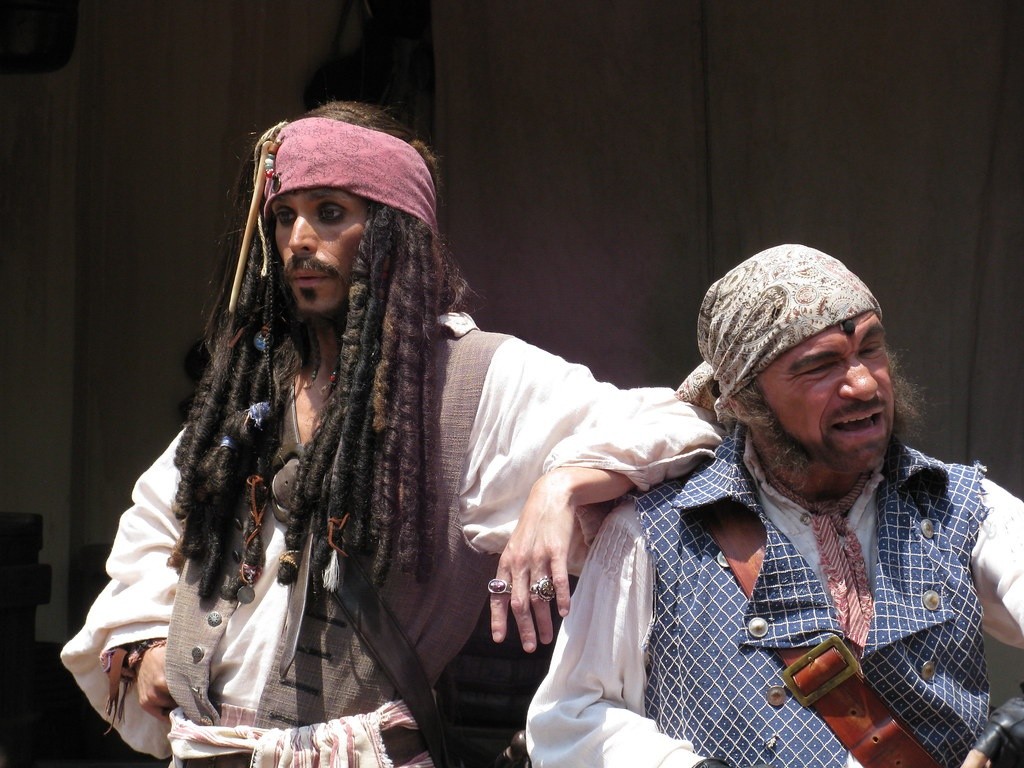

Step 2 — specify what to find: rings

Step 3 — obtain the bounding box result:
[529,576,556,601]
[486,579,512,595]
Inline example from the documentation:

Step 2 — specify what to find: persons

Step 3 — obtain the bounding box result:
[529,240,1024,768]
[58,99,724,766]
